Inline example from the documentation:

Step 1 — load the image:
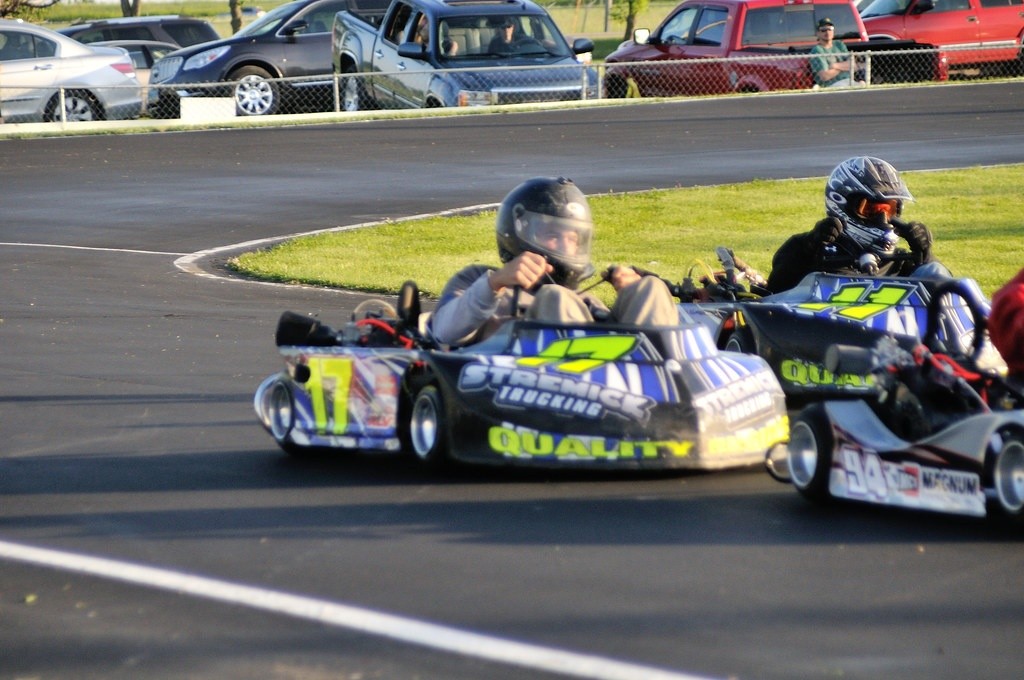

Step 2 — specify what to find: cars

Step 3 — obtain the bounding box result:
[85,38,183,119]
[151,1,395,118]
[857,0,1024,73]
[0,18,145,125]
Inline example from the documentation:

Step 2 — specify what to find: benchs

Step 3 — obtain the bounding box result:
[396,27,495,56]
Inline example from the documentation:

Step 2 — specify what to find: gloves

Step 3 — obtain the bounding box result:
[894,221,932,264]
[805,216,844,251]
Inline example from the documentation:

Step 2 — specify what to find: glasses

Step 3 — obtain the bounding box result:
[416,22,427,36]
[819,26,834,33]
[498,23,513,30]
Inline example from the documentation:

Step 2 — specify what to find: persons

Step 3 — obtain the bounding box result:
[988,267,1024,392]
[811,18,863,87]
[760,155,951,302]
[429,178,678,352]
[420,15,556,59]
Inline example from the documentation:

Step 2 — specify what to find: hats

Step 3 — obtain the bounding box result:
[495,16,515,25]
[816,18,834,30]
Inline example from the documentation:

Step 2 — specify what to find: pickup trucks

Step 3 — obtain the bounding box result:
[330,1,601,116]
[601,2,949,99]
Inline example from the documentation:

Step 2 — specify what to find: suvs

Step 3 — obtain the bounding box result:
[39,13,223,55]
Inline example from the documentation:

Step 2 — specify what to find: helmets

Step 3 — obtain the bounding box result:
[825,156,915,256]
[496,177,593,289]
